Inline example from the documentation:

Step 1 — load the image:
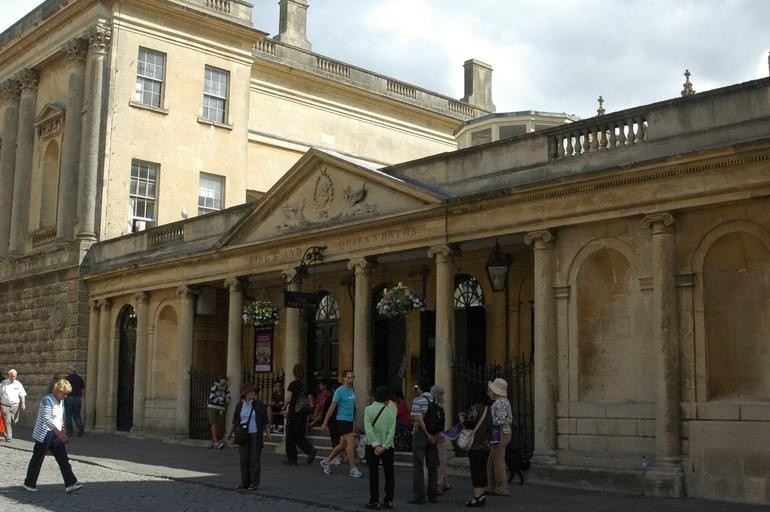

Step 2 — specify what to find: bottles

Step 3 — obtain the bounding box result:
[641,456,647,471]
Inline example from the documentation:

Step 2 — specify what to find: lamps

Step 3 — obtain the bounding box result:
[485,238,511,292]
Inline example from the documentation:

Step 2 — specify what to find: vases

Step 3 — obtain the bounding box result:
[392,311,408,317]
[254,324,274,330]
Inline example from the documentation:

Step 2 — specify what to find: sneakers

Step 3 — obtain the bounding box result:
[66,432,72,437]
[348,467,363,478]
[306,448,317,464]
[22,482,39,492]
[78,424,84,436]
[320,459,332,476]
[4,436,12,442]
[483,490,508,496]
[282,460,297,466]
[263,424,284,436]
[330,455,342,465]
[206,442,224,450]
[65,482,84,493]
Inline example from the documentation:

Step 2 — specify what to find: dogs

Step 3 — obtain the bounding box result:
[505,446,532,484]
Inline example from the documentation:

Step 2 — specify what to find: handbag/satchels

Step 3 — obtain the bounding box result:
[456,427,475,452]
[354,425,367,460]
[233,422,250,445]
[487,424,502,446]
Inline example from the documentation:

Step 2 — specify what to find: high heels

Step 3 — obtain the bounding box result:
[467,494,487,505]
[463,494,483,507]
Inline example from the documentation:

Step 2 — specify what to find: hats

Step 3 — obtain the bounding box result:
[487,377,508,397]
[239,381,262,400]
[216,375,228,381]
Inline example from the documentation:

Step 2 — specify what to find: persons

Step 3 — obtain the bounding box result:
[266,381,287,430]
[364,384,398,509]
[64,366,84,437]
[21,379,84,493]
[282,361,411,478]
[231,381,268,491]
[0,368,28,441]
[410,376,517,508]
[208,372,231,448]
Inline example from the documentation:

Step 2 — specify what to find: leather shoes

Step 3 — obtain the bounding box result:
[427,498,438,503]
[380,500,393,510]
[406,498,425,506]
[364,501,381,510]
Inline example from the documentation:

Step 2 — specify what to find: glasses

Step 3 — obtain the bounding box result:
[348,374,355,379]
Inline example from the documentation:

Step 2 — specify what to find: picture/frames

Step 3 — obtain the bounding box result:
[253,330,274,373]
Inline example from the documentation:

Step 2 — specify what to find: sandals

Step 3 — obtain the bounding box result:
[248,483,258,491]
[442,483,452,490]
[235,483,250,489]
[436,487,444,496]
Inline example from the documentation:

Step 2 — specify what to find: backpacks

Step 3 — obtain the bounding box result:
[292,379,312,416]
[420,395,446,436]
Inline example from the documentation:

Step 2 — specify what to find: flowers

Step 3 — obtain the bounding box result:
[242,301,282,330]
[376,281,426,320]
